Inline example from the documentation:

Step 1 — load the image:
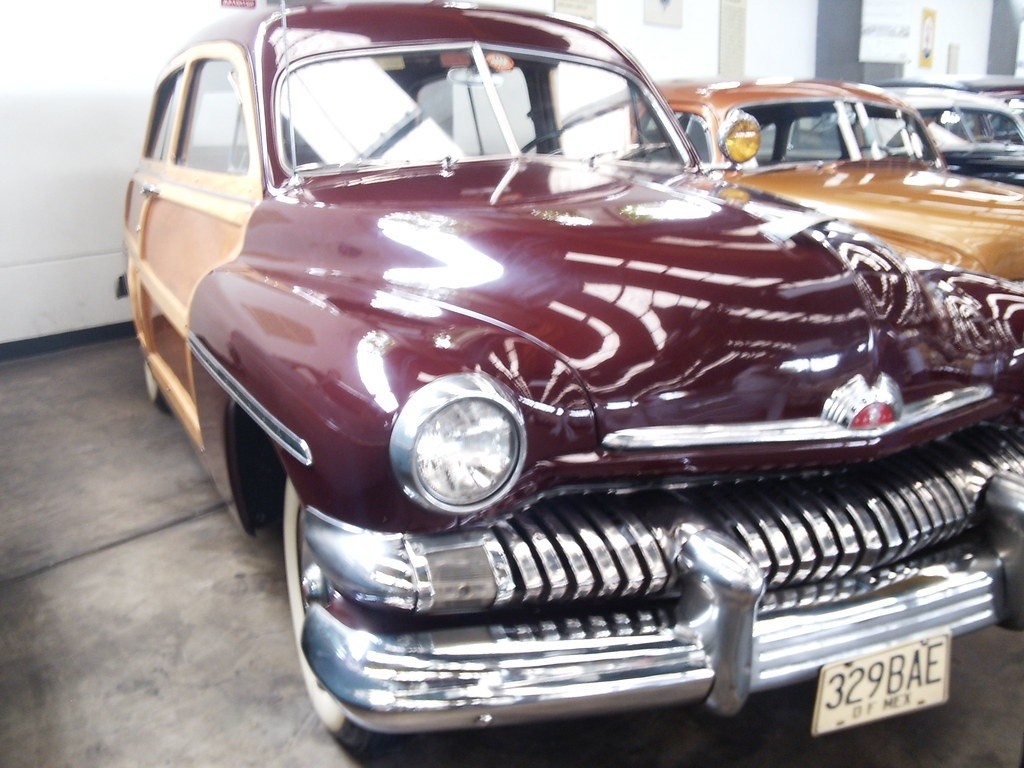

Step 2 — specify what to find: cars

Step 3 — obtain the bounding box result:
[617,78,1024,288]
[119,0,1023,767]
[803,82,1023,185]
